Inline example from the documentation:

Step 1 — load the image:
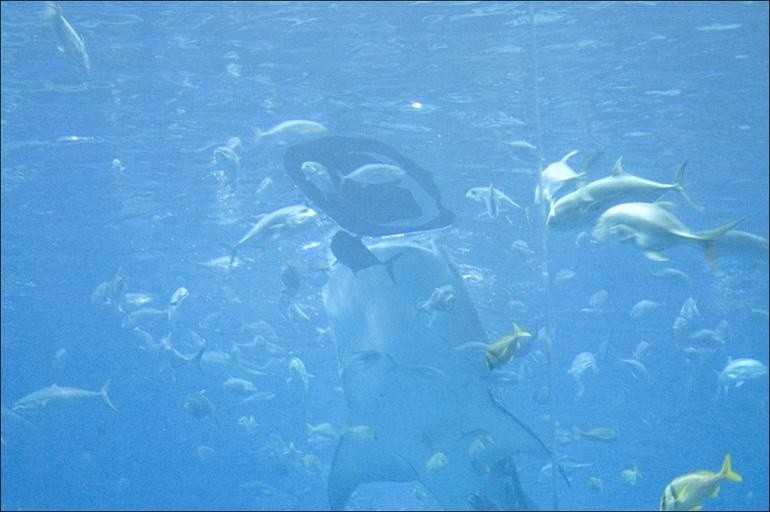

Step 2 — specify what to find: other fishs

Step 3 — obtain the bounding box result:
[0,1,768,511]
